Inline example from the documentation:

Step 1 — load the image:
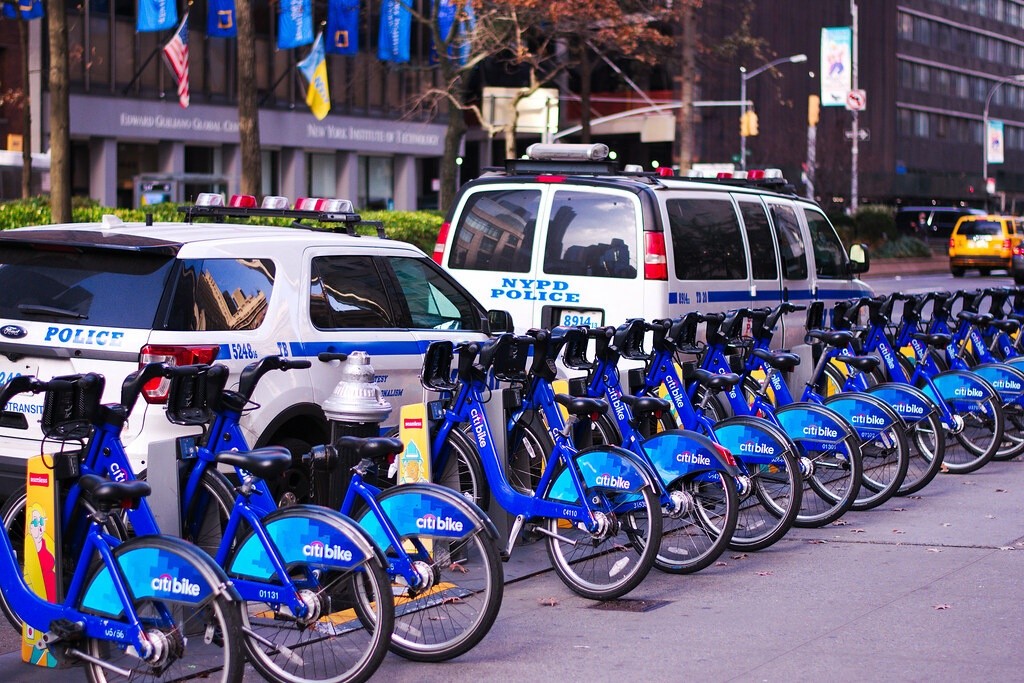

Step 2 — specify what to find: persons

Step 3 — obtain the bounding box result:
[911,212,928,243]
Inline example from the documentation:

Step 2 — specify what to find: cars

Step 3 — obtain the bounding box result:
[0,193,515,551]
[1013,241,1024,286]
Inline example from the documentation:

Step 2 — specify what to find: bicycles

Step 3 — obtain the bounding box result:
[407,283,1024,600]
[320,436,505,662]
[146,352,395,683]
[0,359,248,683]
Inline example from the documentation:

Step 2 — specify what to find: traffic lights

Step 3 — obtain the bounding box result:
[746,110,760,137]
[968,183,977,199]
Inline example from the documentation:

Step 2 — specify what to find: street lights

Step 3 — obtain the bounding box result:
[982,75,1023,212]
[741,53,807,173]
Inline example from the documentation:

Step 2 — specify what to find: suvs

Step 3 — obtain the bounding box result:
[949,217,1024,277]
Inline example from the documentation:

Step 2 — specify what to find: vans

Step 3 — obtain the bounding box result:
[893,206,991,250]
[420,143,879,395]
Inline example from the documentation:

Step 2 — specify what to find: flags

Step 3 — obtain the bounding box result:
[297,29,332,121]
[160,11,191,109]
